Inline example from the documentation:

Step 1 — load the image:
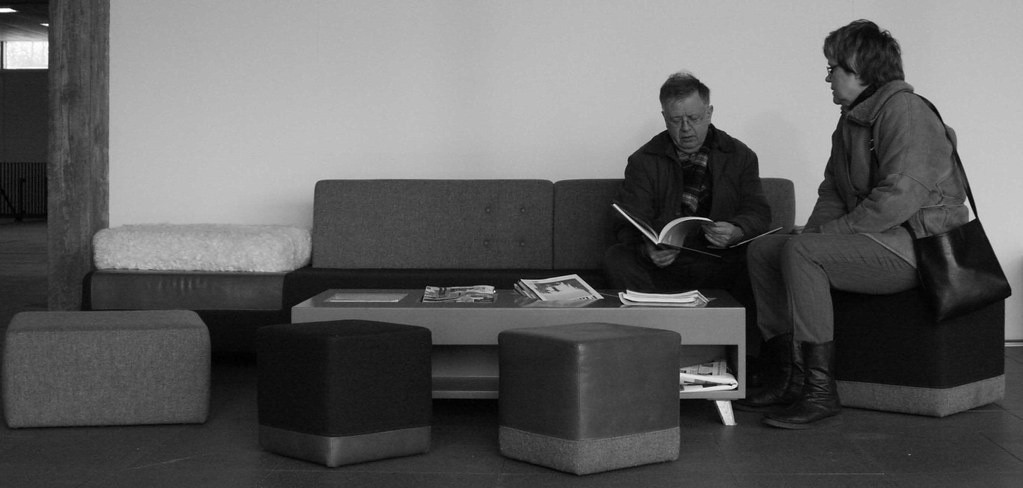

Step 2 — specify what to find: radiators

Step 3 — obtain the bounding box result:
[0,162,47,215]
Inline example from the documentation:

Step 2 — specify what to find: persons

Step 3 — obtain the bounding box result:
[729,20,971,428]
[604,72,775,382]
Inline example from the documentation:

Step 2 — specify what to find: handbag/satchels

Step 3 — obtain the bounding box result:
[914,218,1011,324]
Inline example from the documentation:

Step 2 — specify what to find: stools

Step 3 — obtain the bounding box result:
[87,220,316,362]
[9,309,213,428]
[830,287,1005,419]
[496,323,680,476]
[257,317,430,469]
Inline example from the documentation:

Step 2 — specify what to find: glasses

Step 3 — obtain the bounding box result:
[663,105,709,127]
[826,63,840,79]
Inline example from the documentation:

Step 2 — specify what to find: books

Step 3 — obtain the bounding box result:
[325,292,407,304]
[610,192,782,305]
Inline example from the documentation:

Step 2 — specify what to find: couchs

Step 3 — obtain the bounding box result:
[302,176,802,393]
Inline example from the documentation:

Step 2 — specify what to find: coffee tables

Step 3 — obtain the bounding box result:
[290,289,746,426]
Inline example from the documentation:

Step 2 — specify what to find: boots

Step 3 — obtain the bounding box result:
[731,333,794,412]
[761,340,843,429]
